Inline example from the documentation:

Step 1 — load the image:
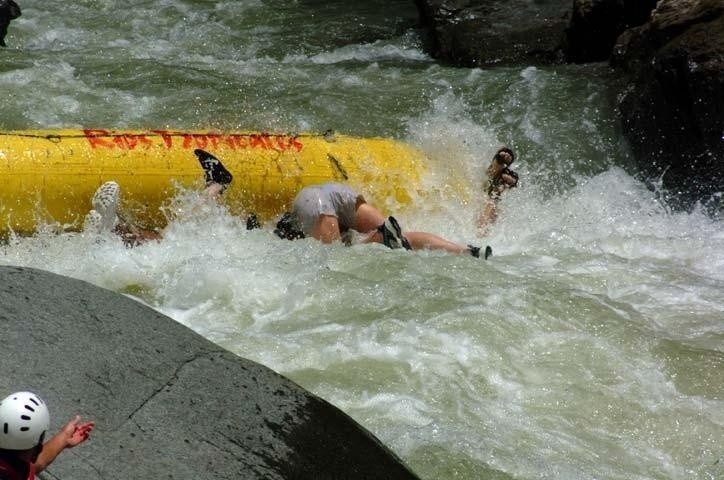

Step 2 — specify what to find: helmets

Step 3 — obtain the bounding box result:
[0,392,50,451]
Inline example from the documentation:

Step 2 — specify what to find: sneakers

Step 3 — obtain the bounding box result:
[377,215,411,250]
[194,148,232,185]
[246,212,260,230]
[83,181,121,235]
[467,244,492,260]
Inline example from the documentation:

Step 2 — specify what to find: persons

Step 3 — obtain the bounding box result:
[0,389,96,479]
[163,147,261,237]
[78,180,168,250]
[465,145,519,240]
[276,181,494,263]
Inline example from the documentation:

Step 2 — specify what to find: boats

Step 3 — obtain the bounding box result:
[0,125,472,243]
